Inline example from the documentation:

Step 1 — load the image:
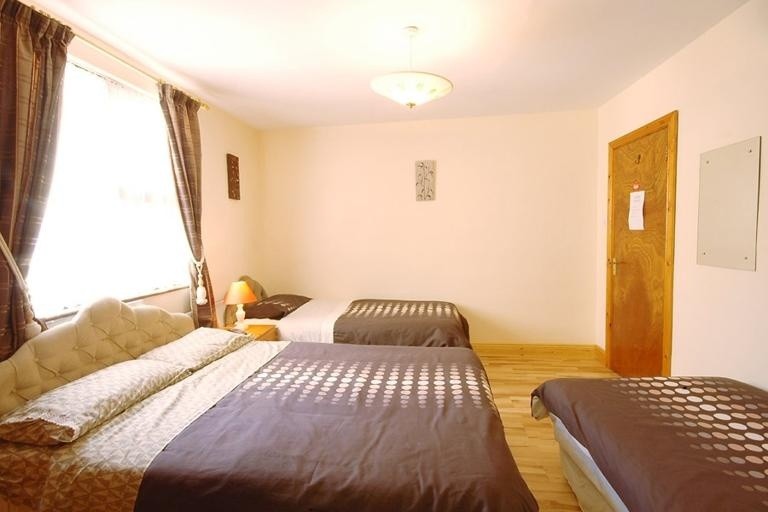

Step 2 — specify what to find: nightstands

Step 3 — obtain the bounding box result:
[224,324,278,342]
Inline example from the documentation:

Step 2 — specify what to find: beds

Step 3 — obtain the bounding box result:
[0,300,536,509]
[530,376,768,511]
[224,275,471,346]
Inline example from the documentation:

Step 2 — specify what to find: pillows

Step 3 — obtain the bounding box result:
[0,326,252,449]
[244,295,312,321]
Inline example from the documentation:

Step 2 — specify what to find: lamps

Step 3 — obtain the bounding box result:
[369,25,454,114]
[221,281,258,330]
